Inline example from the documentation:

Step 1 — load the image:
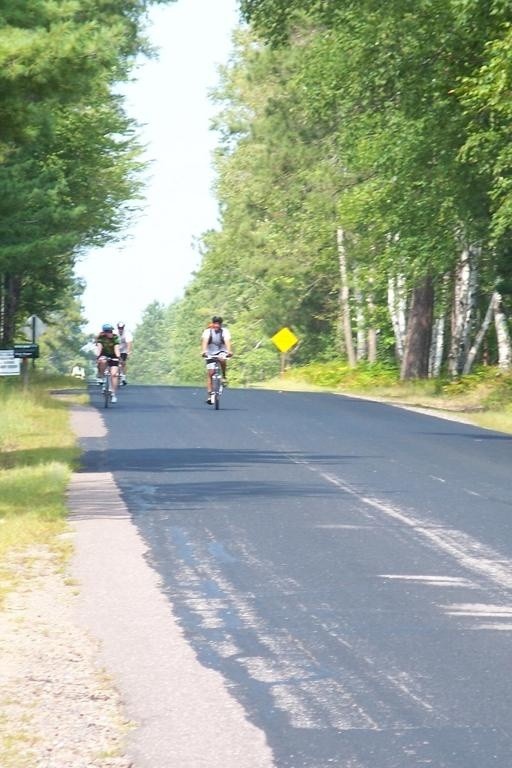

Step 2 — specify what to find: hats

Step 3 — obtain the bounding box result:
[211,316,223,322]
[118,322,125,328]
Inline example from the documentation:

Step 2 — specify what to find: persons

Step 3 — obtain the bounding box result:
[200,314,234,405]
[93,323,122,405]
[114,320,132,386]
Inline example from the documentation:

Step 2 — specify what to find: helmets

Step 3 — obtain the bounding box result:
[102,324,114,330]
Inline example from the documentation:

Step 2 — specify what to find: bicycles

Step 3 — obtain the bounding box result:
[200,351,230,410]
[96,355,125,408]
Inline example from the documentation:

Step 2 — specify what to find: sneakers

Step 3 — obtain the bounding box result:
[206,394,212,406]
[93,374,127,386]
[221,376,227,387]
[110,394,118,405]
